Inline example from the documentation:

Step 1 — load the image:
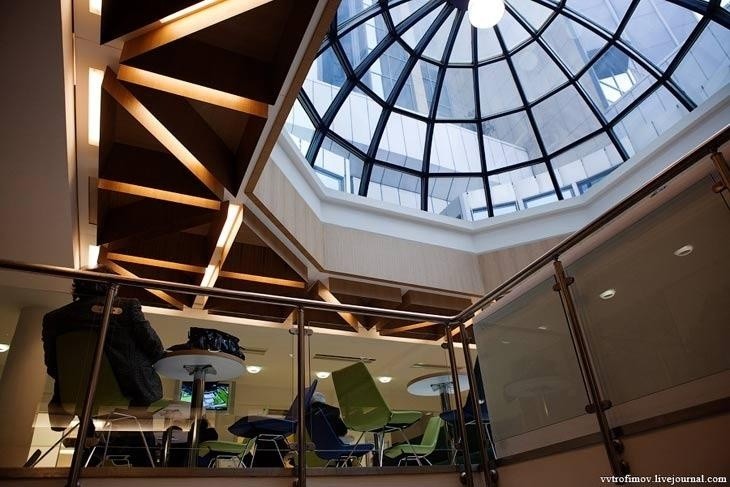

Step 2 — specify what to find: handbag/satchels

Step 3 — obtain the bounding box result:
[187,327,246,360]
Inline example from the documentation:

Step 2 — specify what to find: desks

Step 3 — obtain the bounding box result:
[406,372,472,468]
[153,349,246,468]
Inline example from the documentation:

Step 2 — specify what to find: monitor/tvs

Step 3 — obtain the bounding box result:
[178,380,233,416]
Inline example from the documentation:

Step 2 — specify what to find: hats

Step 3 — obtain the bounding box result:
[312,391,327,402]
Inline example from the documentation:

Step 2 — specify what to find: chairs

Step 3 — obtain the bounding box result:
[385,416,442,466]
[305,404,374,468]
[228,379,317,468]
[200,404,269,469]
[332,361,423,467]
[439,354,498,465]
[56,331,171,467]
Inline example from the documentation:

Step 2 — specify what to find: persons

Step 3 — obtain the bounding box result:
[311,391,352,468]
[188,418,219,467]
[41,266,165,466]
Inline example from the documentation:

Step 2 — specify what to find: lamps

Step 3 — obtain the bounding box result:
[314,372,331,378]
[465,0,505,28]
[377,376,392,383]
[246,366,262,374]
